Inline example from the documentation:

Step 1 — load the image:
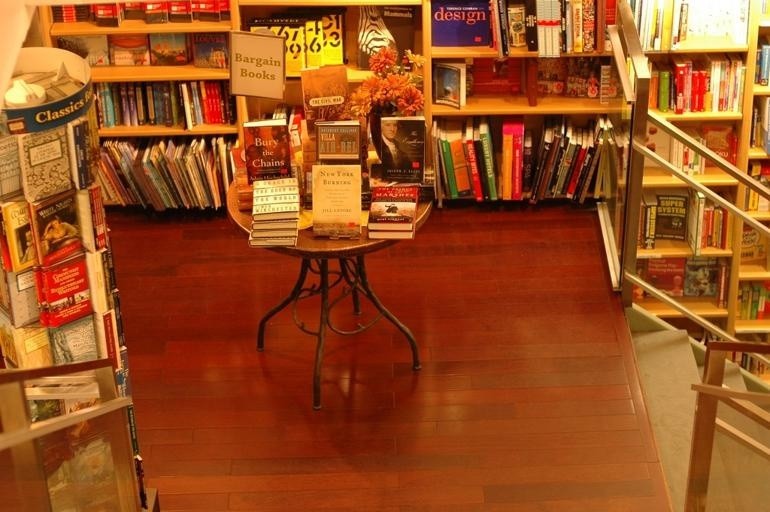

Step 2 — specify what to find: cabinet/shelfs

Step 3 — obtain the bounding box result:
[29,0,620,210]
[0,1,163,512]
[615,0,770,375]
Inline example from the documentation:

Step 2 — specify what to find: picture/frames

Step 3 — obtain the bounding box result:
[228,31,285,101]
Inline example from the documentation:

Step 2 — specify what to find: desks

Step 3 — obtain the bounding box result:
[224,170,432,411]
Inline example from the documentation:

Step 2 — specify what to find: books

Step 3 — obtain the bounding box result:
[634,248,770,380]
